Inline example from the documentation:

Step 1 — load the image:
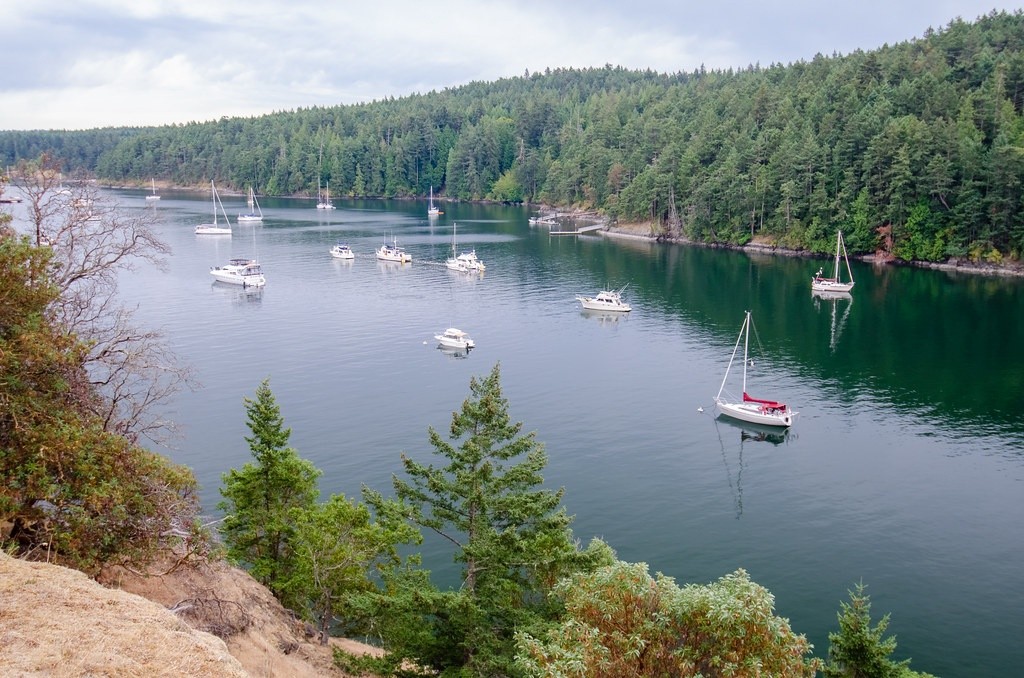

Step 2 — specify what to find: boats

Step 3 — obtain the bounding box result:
[328,244,353,260]
[3,197,24,204]
[577,284,636,313]
[429,328,480,357]
[213,258,265,291]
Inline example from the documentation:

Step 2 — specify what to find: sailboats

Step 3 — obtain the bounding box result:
[807,292,855,354]
[143,177,161,204]
[811,230,854,301]
[236,185,265,223]
[193,180,233,236]
[714,310,796,426]
[716,413,793,522]
[437,223,486,273]
[375,231,413,264]
[423,186,442,219]
[316,179,335,208]
